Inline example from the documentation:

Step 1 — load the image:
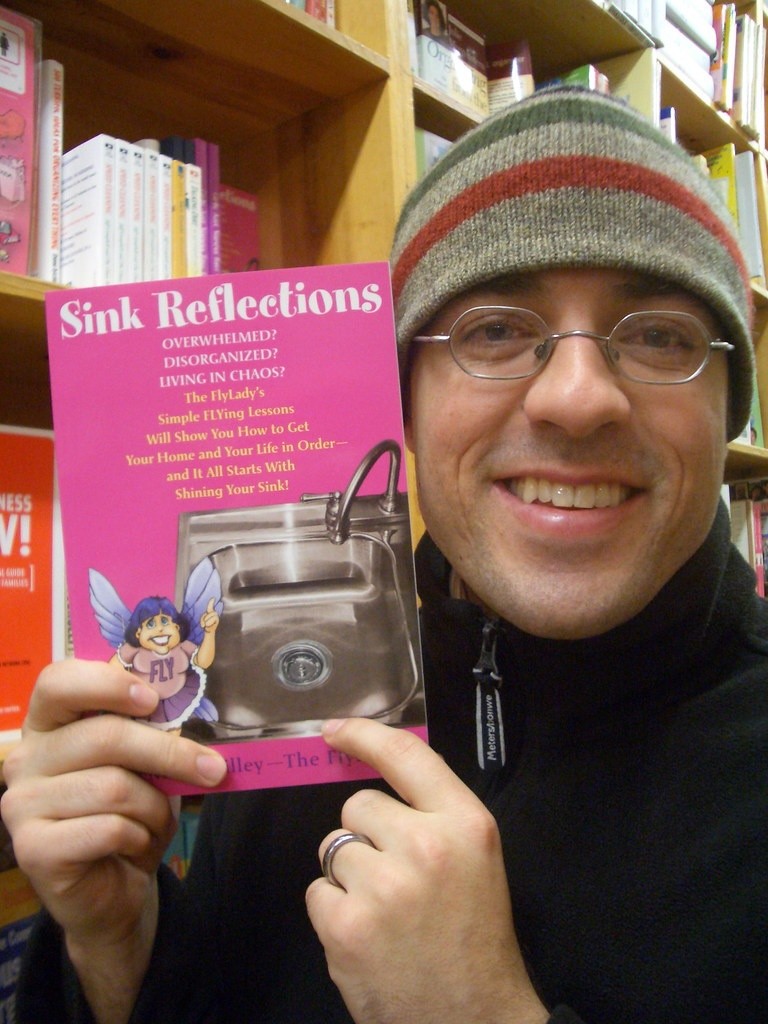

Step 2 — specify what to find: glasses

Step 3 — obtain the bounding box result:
[411,306,735,384]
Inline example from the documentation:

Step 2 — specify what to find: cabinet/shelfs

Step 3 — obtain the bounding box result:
[0,0,768,1024]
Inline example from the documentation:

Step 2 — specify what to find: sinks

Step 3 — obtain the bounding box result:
[174,523,427,747]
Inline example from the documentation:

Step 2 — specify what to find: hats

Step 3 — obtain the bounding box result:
[390,84,755,443]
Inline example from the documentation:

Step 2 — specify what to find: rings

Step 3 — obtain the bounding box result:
[321,834,376,887]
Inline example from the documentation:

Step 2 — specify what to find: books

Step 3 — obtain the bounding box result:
[292,0,336,32]
[596,0,768,292]
[407,0,610,118]
[44,262,429,796]
[1,8,262,291]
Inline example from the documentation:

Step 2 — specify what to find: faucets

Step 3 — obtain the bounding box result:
[299,437,404,546]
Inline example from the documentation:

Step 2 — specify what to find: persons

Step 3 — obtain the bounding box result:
[423,0,452,52]
[1,85,767,1023]
